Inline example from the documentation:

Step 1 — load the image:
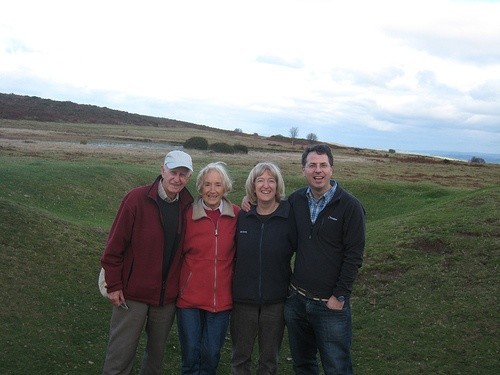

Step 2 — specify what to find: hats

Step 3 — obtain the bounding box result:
[164,150,193,172]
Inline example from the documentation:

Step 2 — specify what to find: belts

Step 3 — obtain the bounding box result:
[290,282,328,301]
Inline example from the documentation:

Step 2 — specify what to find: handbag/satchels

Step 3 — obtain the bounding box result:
[98,268,108,298]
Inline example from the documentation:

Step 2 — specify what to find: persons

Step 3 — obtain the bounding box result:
[103,149,199,375]
[240,144,367,375]
[181,160,243,375]
[232,160,296,374]
